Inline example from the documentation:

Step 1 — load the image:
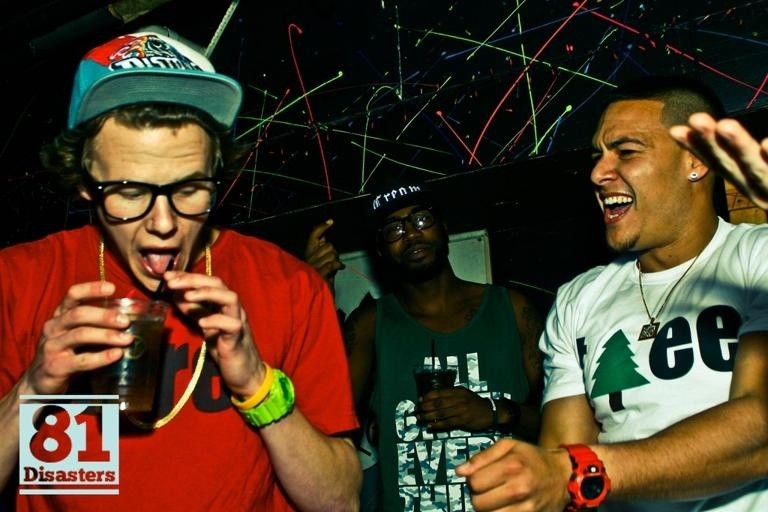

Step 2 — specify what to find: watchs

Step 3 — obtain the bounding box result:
[240,369,296,430]
[558,444,610,509]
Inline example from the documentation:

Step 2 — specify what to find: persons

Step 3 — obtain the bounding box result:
[668,112,768,217]
[1,27,364,511]
[454,76,768,511]
[304,181,546,511]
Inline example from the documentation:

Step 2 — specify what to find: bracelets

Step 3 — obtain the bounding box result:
[231,362,275,407]
[500,396,521,433]
[485,396,498,432]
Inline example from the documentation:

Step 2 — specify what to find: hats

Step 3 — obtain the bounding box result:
[66,27,244,130]
[366,173,445,226]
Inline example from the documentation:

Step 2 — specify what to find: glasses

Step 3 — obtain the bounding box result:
[375,206,438,245]
[79,159,221,225]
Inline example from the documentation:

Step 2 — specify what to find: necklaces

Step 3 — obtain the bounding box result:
[637,258,705,341]
[98,229,213,432]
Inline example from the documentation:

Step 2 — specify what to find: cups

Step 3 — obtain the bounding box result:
[82,297,170,415]
[416,368,460,428]
